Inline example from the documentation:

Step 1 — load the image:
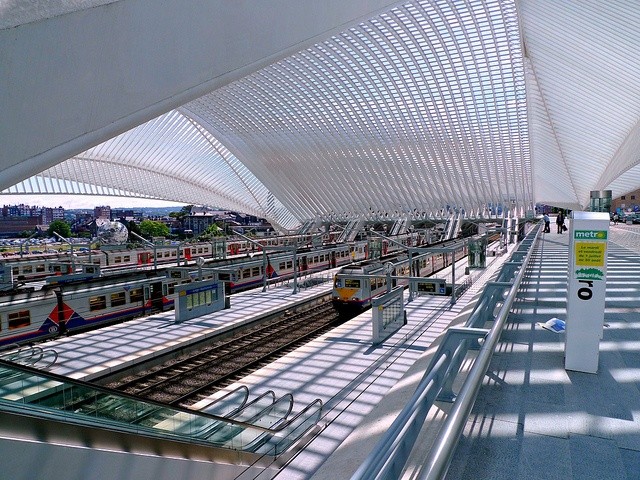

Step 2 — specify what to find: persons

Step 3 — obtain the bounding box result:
[541,212,550,233]
[613,210,620,225]
[556,210,565,233]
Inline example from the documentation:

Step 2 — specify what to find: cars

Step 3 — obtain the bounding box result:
[2,252,16,257]
[67,248,76,253]
[80,247,89,251]
[16,250,30,255]
[48,248,58,254]
[32,250,43,254]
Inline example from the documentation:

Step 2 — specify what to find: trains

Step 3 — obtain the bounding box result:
[330,231,499,319]
[0,232,342,288]
[0,231,444,353]
